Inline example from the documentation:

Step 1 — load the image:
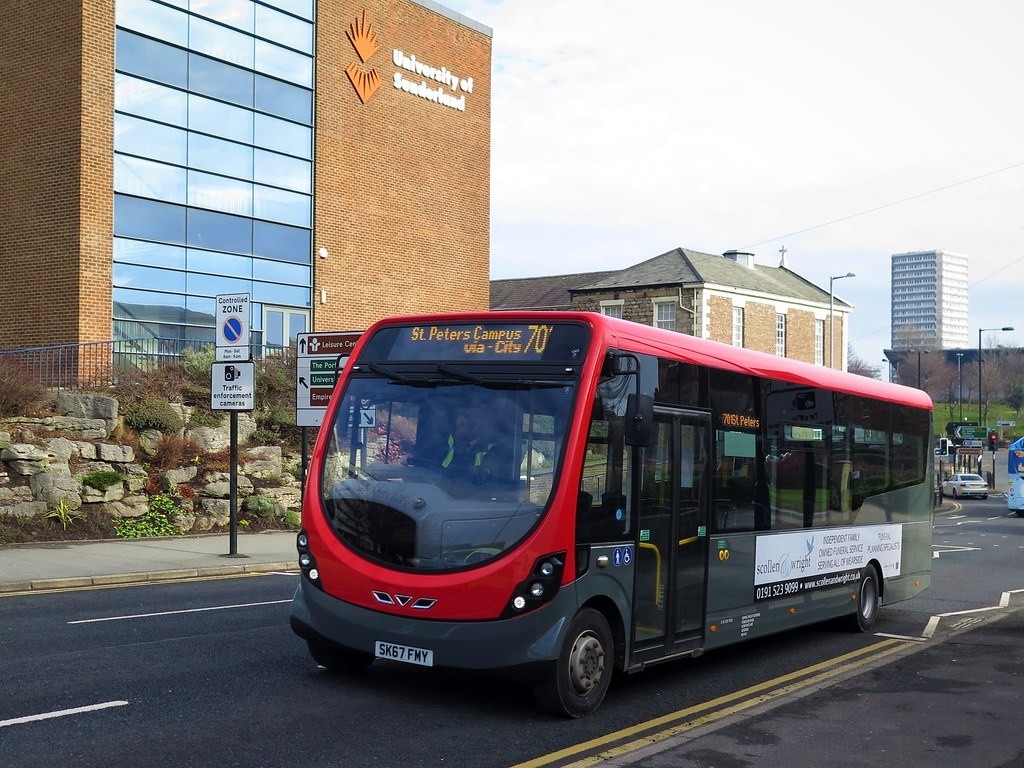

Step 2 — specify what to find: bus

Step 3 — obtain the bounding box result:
[289,314,934,719]
[1007,438,1024,516]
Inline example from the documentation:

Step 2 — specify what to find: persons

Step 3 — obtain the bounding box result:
[436,409,503,487]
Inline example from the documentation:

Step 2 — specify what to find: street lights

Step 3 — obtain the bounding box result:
[988,432,998,451]
[907,347,932,389]
[829,271,856,367]
[941,439,947,456]
[882,358,892,382]
[978,327,1015,426]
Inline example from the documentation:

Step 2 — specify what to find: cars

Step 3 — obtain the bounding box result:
[942,473,989,499]
